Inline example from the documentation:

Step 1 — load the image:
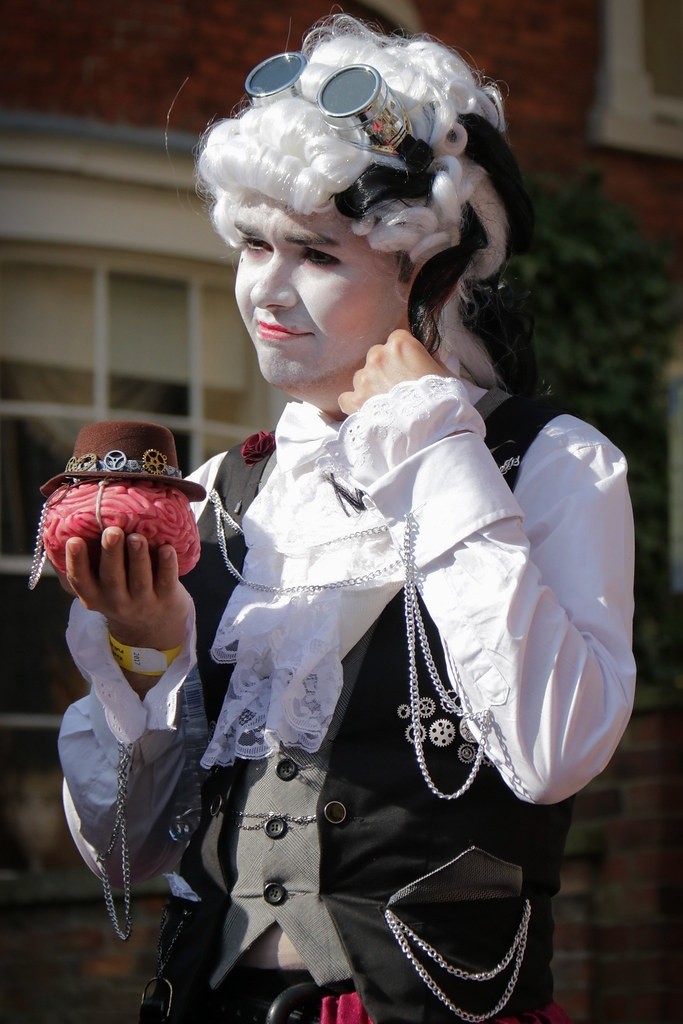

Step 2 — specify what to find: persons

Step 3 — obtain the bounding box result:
[57,12,636,1024]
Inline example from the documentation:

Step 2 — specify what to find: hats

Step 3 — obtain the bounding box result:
[39,418,206,504]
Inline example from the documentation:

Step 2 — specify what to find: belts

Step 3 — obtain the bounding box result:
[181,988,320,1024]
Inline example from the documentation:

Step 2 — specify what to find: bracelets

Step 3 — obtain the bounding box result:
[109,632,184,676]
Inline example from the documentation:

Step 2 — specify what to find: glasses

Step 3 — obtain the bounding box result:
[243,52,434,173]
